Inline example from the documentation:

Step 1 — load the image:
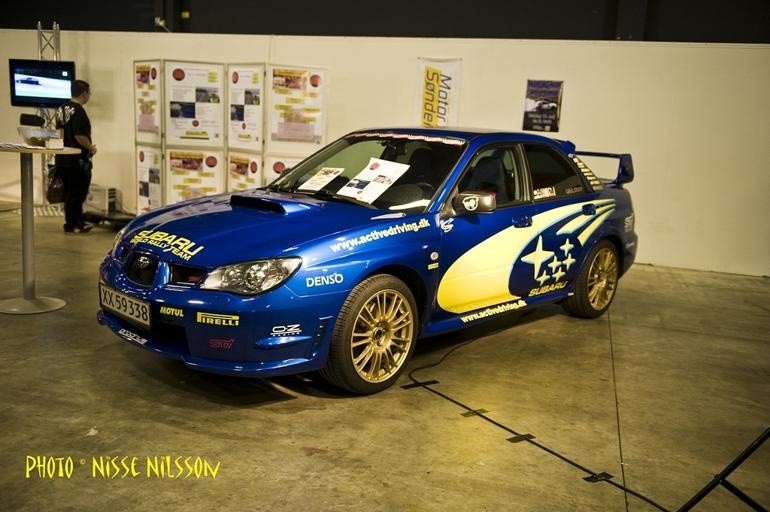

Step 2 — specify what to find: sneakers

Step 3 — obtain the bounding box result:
[64,222,93,234]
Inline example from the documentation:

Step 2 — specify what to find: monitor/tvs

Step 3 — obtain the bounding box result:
[8,58,76,108]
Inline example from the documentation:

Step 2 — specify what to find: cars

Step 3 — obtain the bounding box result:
[534,101,557,110]
[96,127,638,396]
[16,77,39,85]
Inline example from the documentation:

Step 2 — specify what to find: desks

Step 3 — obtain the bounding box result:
[0,142,83,316]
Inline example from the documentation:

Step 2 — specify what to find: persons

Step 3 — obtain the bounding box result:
[54,79,97,234]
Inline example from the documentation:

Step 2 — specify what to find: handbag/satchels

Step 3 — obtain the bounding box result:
[46,164,67,206]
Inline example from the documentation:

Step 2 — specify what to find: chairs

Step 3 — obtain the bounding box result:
[467,157,509,204]
